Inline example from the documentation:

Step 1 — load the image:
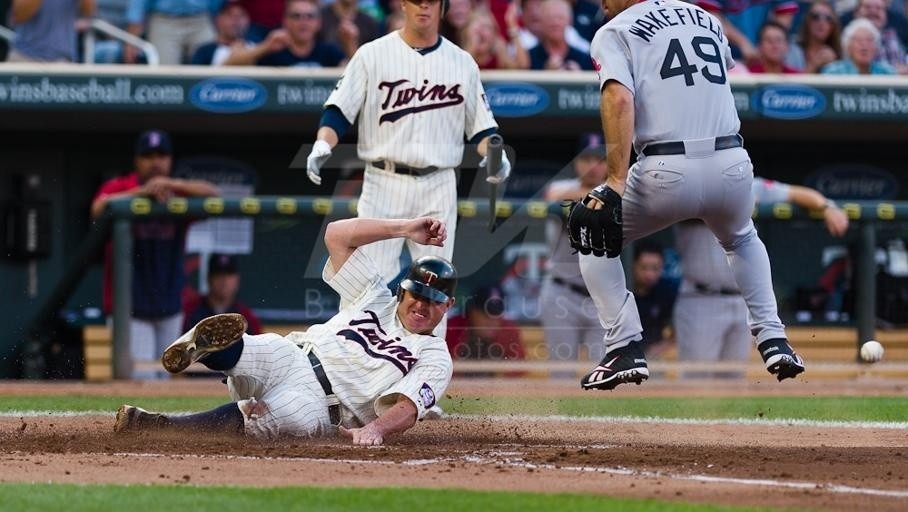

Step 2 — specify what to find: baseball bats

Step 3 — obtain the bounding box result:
[487,135,503,233]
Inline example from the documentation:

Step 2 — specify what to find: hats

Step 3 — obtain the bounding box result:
[132,127,173,157]
[208,253,248,281]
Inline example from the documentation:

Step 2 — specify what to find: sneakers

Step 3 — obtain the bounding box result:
[580,341,649,391]
[113,404,169,436]
[160,313,249,376]
[758,338,806,383]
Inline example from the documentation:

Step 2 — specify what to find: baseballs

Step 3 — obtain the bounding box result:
[862,341,883,362]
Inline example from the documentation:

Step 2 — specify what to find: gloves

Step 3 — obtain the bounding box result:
[478,149,511,184]
[306,140,333,186]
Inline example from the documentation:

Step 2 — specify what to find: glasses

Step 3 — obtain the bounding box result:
[287,10,316,22]
[812,12,833,23]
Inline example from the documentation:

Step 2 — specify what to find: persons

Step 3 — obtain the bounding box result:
[2,0,907,444]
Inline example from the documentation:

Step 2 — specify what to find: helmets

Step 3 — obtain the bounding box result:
[395,255,458,303]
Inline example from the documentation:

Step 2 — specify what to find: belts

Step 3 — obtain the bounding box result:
[372,160,439,176]
[642,134,743,157]
[695,283,740,297]
[297,344,342,426]
[554,277,590,297]
